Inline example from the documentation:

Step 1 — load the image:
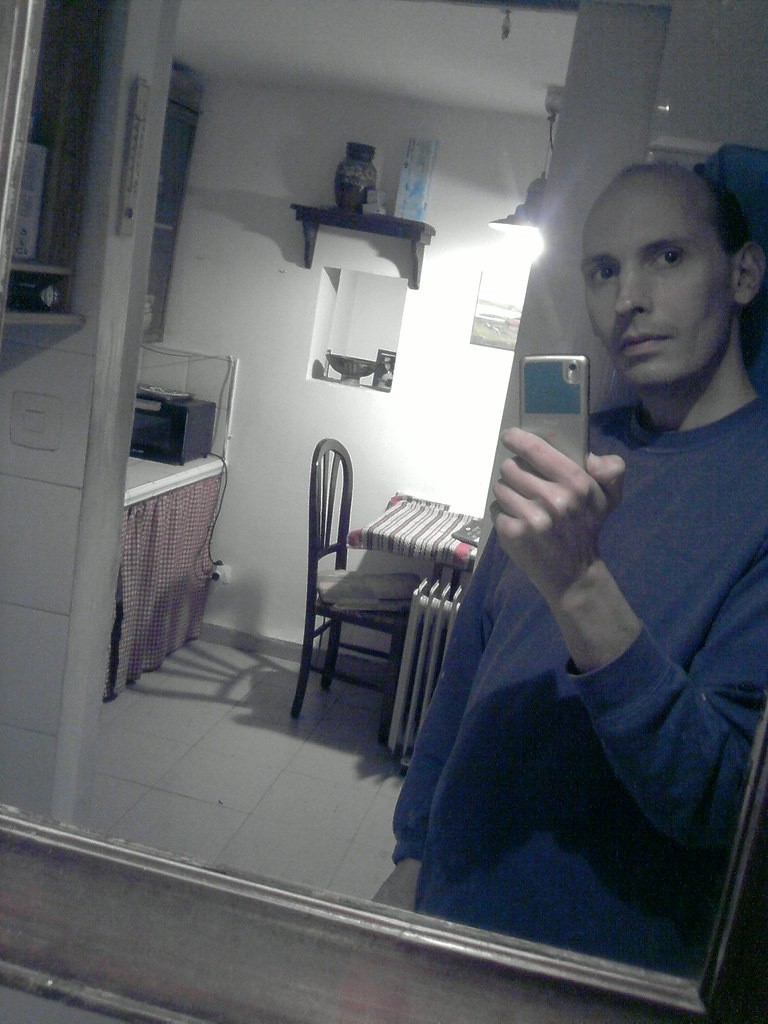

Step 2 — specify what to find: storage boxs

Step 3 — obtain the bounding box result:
[393,135,438,221]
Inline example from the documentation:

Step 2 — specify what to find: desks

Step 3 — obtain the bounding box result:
[343,492,482,606]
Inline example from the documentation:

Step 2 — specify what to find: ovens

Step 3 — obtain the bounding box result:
[132,383,217,467]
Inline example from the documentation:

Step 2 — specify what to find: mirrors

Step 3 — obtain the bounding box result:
[0,1,768,1024]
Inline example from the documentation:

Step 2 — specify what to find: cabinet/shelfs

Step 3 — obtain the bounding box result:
[0,0,88,327]
[138,94,203,344]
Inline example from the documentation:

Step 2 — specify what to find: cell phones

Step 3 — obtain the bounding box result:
[521,355,590,478]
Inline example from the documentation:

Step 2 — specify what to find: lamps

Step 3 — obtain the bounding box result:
[488,86,565,237]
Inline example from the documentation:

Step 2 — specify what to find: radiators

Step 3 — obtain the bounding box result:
[389,576,466,777]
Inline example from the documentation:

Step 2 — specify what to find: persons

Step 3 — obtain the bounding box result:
[373,159,768,980]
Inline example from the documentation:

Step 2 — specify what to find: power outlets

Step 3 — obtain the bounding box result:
[210,563,230,584]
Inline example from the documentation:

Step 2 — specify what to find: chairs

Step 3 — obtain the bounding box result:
[289,437,427,746]
